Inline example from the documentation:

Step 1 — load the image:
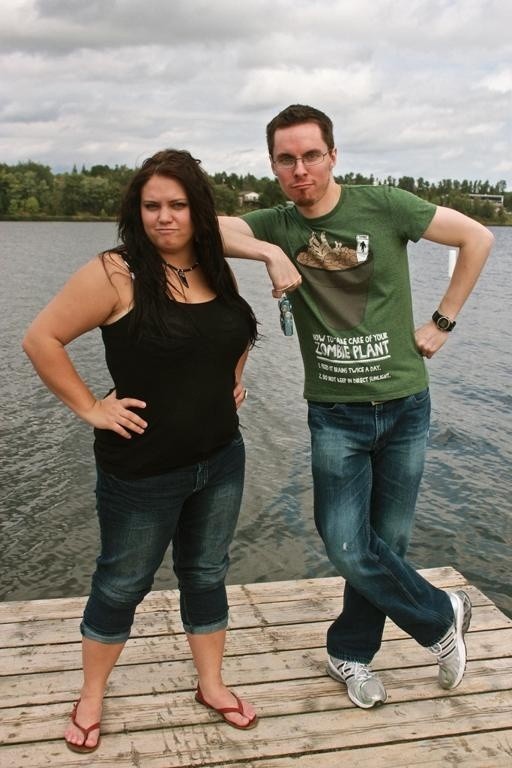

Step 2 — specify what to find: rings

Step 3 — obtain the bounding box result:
[242,390,249,400]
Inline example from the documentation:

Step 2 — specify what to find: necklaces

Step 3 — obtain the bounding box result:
[156,250,202,288]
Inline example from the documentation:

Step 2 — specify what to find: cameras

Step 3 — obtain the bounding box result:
[279,296,294,336]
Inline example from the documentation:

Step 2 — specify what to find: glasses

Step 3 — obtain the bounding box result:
[272,149,330,169]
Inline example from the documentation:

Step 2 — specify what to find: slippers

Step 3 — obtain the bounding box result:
[64,698,102,753]
[195,680,259,730]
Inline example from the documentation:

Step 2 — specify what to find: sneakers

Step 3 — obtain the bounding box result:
[425,591,473,691]
[325,655,389,709]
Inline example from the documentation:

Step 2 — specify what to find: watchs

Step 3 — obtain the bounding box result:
[430,307,458,333]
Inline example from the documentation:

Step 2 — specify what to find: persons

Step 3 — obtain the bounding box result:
[22,147,260,755]
[216,103,496,709]
